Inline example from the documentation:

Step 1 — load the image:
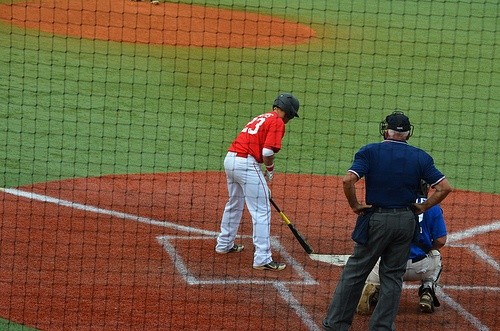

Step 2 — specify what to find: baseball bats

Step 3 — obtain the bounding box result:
[270,198,315,255]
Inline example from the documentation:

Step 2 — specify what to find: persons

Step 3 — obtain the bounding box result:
[356,183,447,315]
[215,93,299,270]
[323,110,451,331]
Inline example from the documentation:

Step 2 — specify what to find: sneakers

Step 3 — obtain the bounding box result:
[229,243,244,252]
[322,318,334,331]
[356,283,376,315]
[253,260,287,271]
[419,292,434,313]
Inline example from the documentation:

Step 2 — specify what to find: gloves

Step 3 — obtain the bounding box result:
[263,169,274,182]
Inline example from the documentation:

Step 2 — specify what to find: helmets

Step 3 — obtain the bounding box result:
[417,179,428,199]
[273,93,299,120]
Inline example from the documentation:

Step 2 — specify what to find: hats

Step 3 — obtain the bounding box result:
[386,114,411,132]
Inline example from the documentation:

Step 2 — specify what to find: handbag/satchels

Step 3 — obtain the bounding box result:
[411,206,420,240]
[351,209,375,244]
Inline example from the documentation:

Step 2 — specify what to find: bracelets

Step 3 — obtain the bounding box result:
[266,164,274,171]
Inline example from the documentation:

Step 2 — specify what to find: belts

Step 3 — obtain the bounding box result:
[234,152,248,157]
[375,207,410,213]
[412,254,428,263]
